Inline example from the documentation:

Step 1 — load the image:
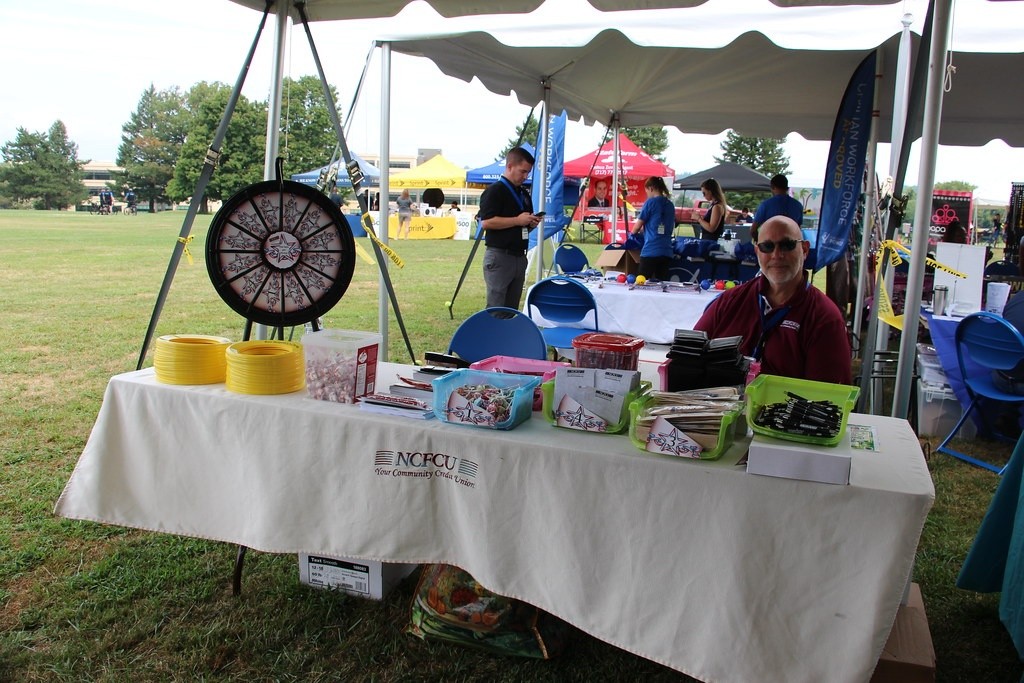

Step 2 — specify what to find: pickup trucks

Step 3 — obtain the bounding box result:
[674,199,754,227]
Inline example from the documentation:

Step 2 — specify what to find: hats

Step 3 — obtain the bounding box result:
[450,201,457,205]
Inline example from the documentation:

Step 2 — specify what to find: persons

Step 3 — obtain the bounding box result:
[124,189,136,208]
[448,201,460,211]
[1002,235,1024,338]
[983,214,1002,248]
[751,175,803,242]
[393,190,413,241]
[734,207,754,225]
[691,178,726,241]
[375,192,380,211]
[1002,205,1011,249]
[475,210,486,236]
[357,190,374,211]
[329,187,343,210]
[100,190,113,215]
[587,180,610,207]
[480,148,543,319]
[692,215,853,391]
[631,177,676,282]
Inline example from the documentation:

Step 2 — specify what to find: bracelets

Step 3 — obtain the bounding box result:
[697,217,702,222]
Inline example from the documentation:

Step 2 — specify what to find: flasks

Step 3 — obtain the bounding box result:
[934,284,948,315]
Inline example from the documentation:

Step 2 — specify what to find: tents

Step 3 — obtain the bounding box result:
[465,134,675,242]
[291,151,488,238]
[220,0,1024,420]
[673,162,772,235]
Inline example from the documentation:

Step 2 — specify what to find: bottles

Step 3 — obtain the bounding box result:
[740,220,742,225]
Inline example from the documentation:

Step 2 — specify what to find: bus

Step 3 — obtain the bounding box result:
[361,187,483,220]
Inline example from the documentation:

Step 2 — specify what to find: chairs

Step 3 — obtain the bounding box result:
[527,276,606,365]
[447,307,547,364]
[554,245,589,275]
[935,311,1024,475]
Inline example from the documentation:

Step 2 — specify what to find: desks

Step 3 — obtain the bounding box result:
[50,342,937,683]
[523,276,738,344]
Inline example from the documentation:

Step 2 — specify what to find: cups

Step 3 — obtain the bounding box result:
[725,234,731,240]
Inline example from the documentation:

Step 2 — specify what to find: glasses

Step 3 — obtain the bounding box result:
[756,239,803,254]
[742,213,747,216]
[703,191,710,196]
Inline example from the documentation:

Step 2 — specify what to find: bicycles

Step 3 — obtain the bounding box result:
[89,201,119,216]
[123,201,137,216]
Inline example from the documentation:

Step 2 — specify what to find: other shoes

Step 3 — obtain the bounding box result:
[404,238,407,240]
[394,237,398,240]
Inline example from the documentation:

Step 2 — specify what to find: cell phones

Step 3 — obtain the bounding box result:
[534,211,547,216]
